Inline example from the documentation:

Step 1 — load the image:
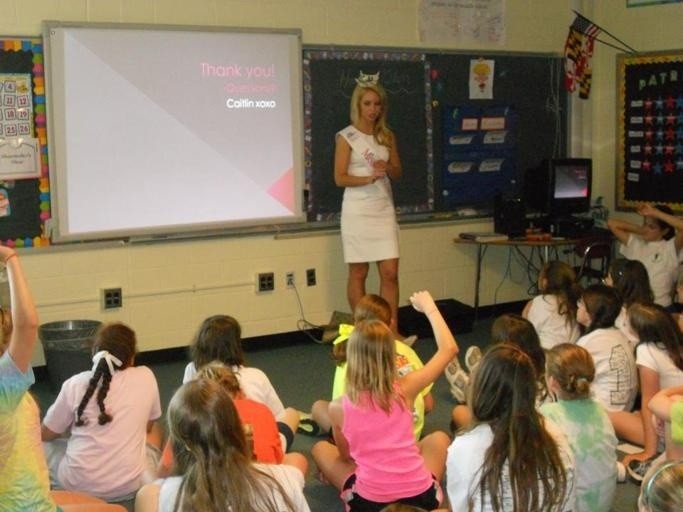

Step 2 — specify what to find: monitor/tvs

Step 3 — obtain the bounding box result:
[525,158,592,215]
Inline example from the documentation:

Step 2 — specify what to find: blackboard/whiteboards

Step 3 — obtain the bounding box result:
[298,47,432,222]
[1,34,50,250]
[430,53,564,223]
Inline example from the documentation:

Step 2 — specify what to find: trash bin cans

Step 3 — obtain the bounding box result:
[38,319,101,394]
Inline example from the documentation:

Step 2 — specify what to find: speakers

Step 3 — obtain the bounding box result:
[495,192,526,234]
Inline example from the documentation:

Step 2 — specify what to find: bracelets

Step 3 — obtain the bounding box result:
[0,252,20,267]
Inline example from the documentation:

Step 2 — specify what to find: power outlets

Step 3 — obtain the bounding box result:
[286,272,295,288]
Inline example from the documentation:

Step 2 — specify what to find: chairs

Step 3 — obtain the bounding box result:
[568,241,612,287]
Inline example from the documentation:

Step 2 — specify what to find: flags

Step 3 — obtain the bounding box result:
[573,16,601,39]
[564,30,595,92]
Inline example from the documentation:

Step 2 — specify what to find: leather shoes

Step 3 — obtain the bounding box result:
[403,334,418,348]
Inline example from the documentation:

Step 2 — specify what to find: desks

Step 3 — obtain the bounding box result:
[453,235,619,323]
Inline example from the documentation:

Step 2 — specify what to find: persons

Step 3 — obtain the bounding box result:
[40,322,166,506]
[312,290,460,511]
[0,245,130,512]
[447,197,683,512]
[333,71,419,351]
[155,314,309,479]
[131,377,315,511]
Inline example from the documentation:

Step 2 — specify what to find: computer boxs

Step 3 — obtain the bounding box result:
[541,213,595,236]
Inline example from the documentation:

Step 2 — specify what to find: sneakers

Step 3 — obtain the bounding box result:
[465,344,484,373]
[444,354,470,405]
